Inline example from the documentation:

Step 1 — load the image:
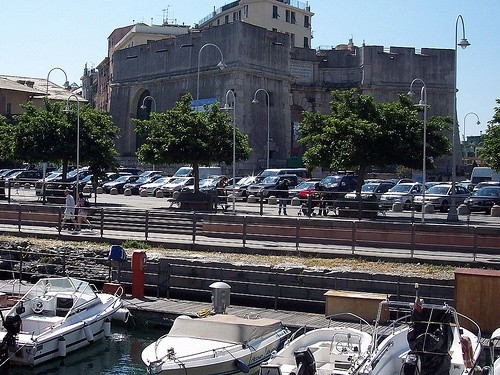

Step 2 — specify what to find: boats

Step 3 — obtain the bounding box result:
[0,277,123,367]
[259,313,374,375]
[140,310,292,375]
[480,325,500,375]
[354,299,483,375]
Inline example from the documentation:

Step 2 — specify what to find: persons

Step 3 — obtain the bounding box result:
[298,192,327,217]
[173,189,180,205]
[275,179,289,215]
[75,192,95,231]
[55,188,75,230]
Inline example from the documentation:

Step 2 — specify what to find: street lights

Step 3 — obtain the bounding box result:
[442,14,472,223]
[46,67,70,99]
[252,89,270,169]
[140,96,156,171]
[407,78,428,228]
[196,42,227,117]
[463,112,481,170]
[61,95,80,206]
[220,89,237,216]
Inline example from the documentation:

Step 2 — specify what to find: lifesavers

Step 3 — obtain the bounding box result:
[461,336,475,368]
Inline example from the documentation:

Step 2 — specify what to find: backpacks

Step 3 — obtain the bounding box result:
[81,201,90,210]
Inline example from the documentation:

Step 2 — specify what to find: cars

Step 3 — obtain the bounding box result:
[0,161,493,213]
[464,186,500,216]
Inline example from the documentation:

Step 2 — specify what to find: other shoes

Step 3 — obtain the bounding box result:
[91,225,95,230]
[56,227,61,231]
[71,230,77,233]
[77,230,81,231]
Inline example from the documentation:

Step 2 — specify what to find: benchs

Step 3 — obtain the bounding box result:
[40,196,67,204]
[21,314,63,331]
[167,199,210,209]
